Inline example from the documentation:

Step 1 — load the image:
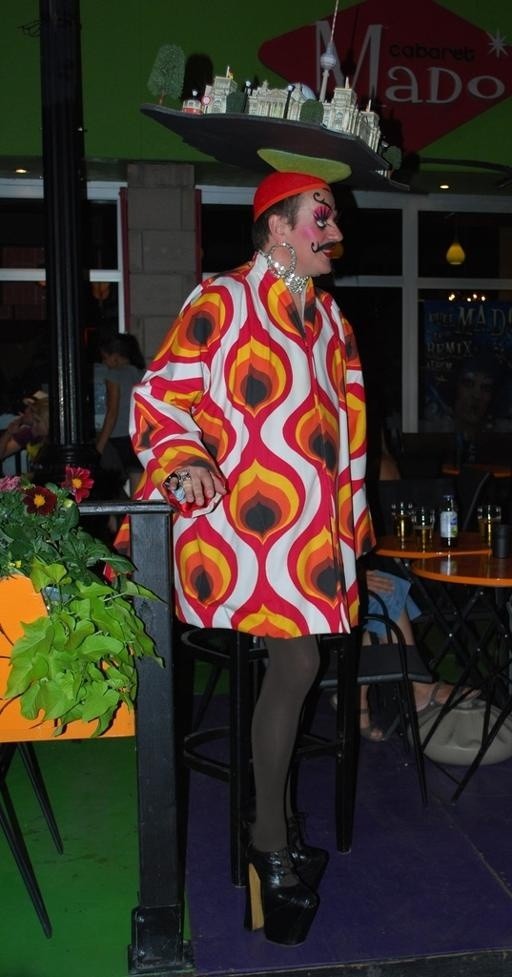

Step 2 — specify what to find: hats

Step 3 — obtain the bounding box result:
[254,171,326,218]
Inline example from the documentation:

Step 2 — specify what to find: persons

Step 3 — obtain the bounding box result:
[357,564,482,745]
[126,170,381,947]
[91,331,145,554]
[0,396,50,461]
[419,348,511,436]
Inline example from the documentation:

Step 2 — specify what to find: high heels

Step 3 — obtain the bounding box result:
[243,843,319,948]
[242,809,328,891]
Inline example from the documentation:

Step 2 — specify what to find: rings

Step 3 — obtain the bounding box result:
[167,472,180,484]
[177,469,193,482]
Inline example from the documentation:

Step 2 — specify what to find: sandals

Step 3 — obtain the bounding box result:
[413,679,482,726]
[332,693,385,744]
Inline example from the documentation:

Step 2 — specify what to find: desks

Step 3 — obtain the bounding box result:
[376,446,512,809]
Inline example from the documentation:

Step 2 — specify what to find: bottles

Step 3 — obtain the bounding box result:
[385,494,500,546]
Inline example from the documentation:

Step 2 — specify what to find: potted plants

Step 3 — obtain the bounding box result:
[4,554,169,740]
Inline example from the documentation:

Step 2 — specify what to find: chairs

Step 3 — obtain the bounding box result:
[172,551,436,889]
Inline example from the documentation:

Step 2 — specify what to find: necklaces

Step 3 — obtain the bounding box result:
[258,250,310,295]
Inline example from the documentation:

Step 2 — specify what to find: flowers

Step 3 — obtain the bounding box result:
[0,462,139,588]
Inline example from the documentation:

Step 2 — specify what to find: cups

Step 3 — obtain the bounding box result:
[493,525,509,558]
